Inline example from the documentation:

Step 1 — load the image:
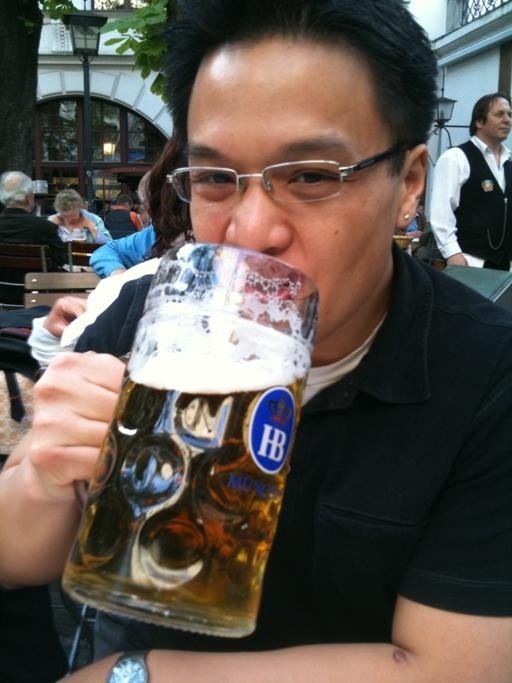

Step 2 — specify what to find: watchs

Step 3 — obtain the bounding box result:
[106,647,151,683]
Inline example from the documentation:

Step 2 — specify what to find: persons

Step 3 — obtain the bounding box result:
[0,1,512,683]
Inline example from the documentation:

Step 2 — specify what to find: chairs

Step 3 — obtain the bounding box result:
[0,240,109,422]
[64,604,99,677]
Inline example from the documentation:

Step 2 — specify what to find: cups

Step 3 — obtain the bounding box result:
[57,243,320,642]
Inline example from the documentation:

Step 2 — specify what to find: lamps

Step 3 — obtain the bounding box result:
[102,138,116,159]
[431,87,475,149]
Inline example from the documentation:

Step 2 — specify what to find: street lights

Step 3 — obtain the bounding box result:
[432,86,457,148]
[58,5,109,201]
[101,135,118,200]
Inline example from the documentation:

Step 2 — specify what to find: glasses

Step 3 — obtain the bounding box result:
[162,140,407,210]
